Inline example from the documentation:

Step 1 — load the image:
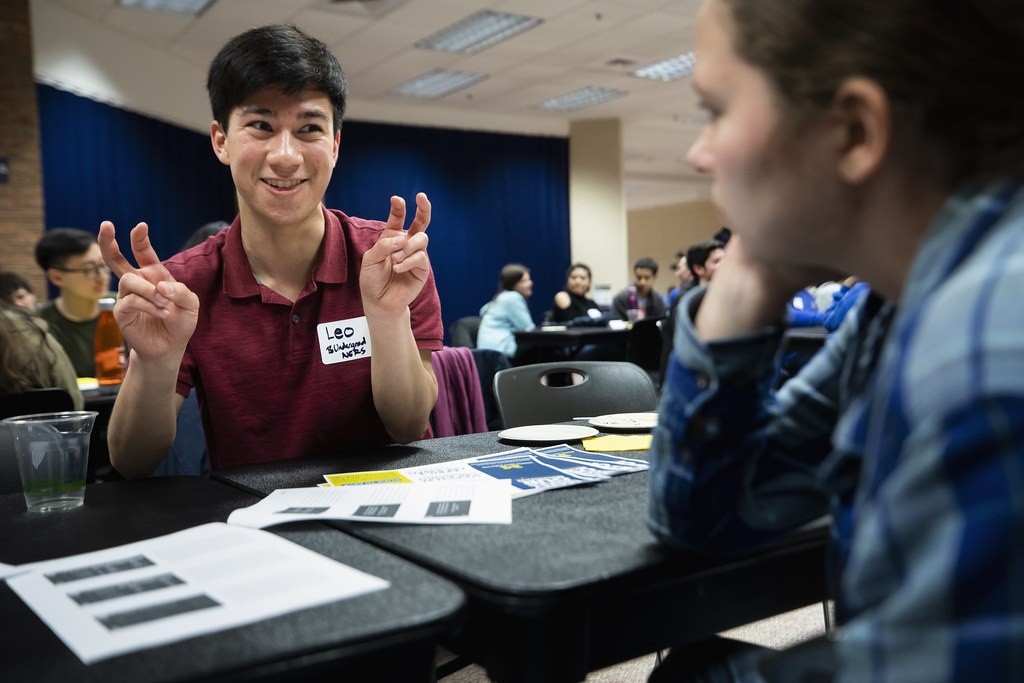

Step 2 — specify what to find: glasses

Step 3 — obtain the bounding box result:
[53,261,109,278]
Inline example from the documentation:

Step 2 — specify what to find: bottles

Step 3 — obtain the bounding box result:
[94,297,129,395]
[625,287,638,311]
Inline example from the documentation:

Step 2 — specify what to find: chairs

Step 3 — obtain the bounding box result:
[0,318,657,683]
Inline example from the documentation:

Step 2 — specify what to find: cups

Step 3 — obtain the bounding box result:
[1,411,98,515]
[627,310,639,324]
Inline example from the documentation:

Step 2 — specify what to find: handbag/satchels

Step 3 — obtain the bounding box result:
[0,305,84,411]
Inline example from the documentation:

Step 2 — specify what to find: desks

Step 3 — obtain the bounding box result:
[512,323,656,363]
[213,411,833,683]
[0,473,468,683]
[786,326,830,351]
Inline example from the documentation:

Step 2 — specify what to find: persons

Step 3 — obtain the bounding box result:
[551,263,599,322]
[31,229,111,378]
[661,226,871,375]
[0,298,85,411]
[0,273,37,311]
[610,257,666,322]
[476,265,536,367]
[97,23,444,478]
[647,0,1024,683]
